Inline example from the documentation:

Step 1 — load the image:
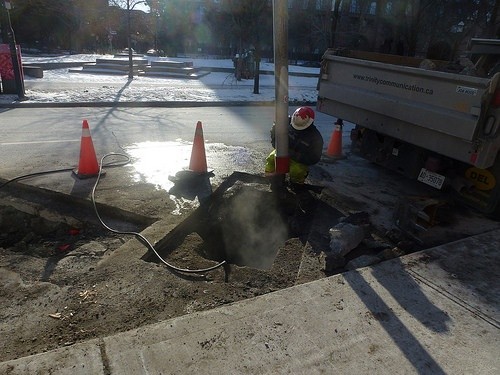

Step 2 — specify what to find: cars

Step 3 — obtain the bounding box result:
[120,47,136,54]
[147,49,164,56]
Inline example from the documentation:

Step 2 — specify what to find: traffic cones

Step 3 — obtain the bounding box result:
[183,121,214,175]
[323,125,347,159]
[72,119,106,178]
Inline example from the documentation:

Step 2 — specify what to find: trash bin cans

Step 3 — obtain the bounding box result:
[232,49,258,82]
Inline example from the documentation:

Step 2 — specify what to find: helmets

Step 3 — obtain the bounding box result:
[291,107,315,130]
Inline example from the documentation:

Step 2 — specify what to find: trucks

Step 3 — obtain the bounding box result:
[317,37,500,219]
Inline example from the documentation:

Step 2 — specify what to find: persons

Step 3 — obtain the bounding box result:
[264,107,324,193]
